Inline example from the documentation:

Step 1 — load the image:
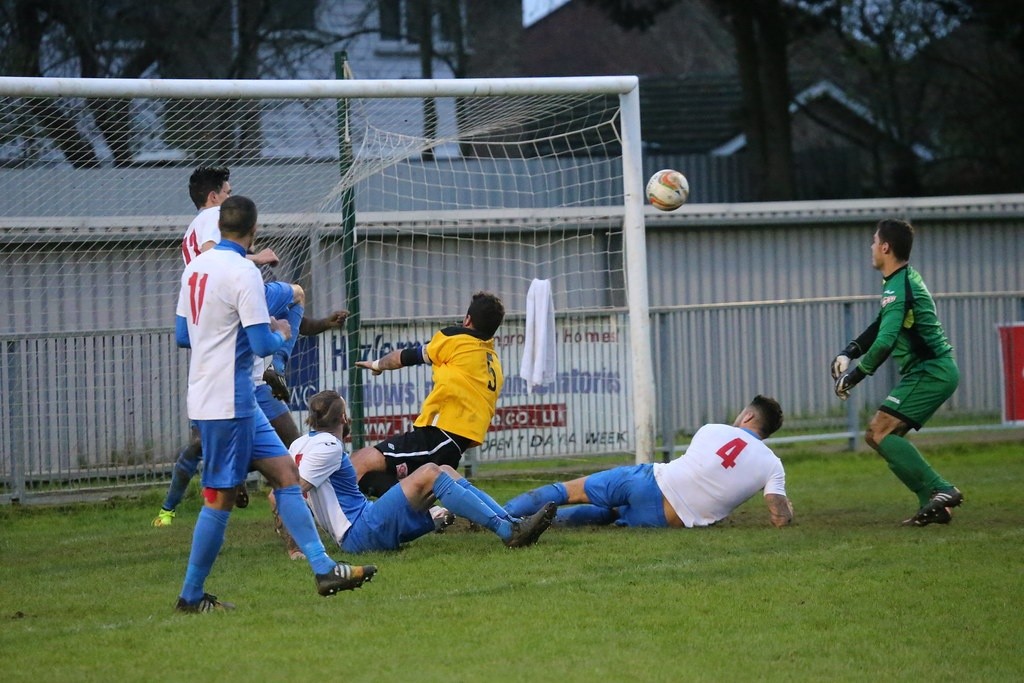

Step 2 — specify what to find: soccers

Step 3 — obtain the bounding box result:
[645,168,690,211]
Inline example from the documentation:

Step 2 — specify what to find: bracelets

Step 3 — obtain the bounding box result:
[372,360,380,371]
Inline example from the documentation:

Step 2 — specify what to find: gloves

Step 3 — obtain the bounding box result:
[831,340,862,380]
[834,367,867,400]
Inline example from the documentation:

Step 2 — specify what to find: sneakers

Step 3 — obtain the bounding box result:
[315,560,377,596]
[173,593,235,614]
[235,485,249,508]
[262,364,290,402]
[898,506,953,528]
[915,486,964,522]
[502,501,557,548]
[433,507,456,534]
[151,508,175,528]
[470,520,488,532]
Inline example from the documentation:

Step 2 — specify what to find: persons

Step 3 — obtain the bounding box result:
[831,218,963,525]
[268,390,557,561]
[470,395,794,532]
[181,167,306,510]
[152,310,351,527]
[171,195,378,615]
[349,291,504,533]
